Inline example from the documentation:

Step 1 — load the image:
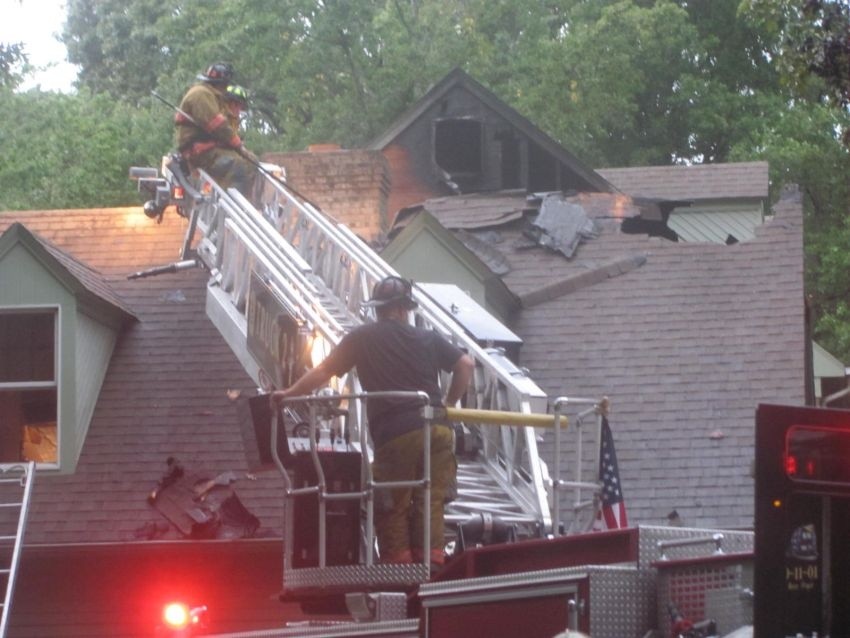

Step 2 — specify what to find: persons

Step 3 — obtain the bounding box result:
[175,62,275,227]
[270,276,475,596]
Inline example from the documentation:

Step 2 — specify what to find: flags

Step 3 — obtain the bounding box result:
[592,422,627,532]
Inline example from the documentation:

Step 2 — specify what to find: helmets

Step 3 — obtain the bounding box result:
[196,62,236,84]
[359,276,417,309]
[224,83,247,105]
